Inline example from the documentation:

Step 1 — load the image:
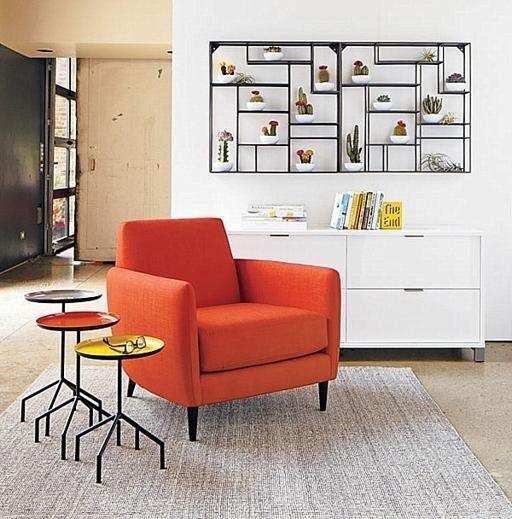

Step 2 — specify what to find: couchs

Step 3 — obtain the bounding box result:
[107,218,341,442]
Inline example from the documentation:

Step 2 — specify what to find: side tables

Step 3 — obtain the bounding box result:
[34,313,121,461]
[21,289,103,436]
[72,335,166,484]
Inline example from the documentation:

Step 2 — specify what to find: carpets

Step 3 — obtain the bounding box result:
[0,365,511,518]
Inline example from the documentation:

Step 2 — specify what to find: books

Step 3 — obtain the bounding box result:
[330,191,403,231]
[239,204,308,231]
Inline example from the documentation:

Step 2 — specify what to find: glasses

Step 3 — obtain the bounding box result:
[104,336,146,354]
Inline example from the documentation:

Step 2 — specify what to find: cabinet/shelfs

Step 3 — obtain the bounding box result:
[225,231,346,347]
[347,228,486,364]
[208,40,471,175]
